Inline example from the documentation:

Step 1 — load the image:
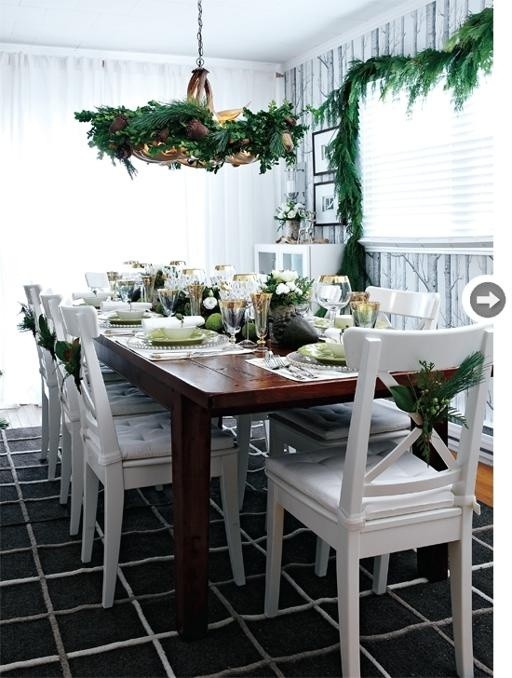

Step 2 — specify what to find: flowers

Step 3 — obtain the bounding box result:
[274,197,310,220]
[259,267,314,305]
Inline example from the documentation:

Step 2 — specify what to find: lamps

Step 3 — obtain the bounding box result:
[75,3,314,179]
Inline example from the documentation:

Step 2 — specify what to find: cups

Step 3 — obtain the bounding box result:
[347,291,370,304]
[351,301,379,329]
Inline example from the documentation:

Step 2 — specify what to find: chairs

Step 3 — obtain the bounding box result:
[257,322,494,675]
[22,281,248,611]
[214,285,441,596]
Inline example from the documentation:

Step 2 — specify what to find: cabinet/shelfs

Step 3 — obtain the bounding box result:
[254,242,347,281]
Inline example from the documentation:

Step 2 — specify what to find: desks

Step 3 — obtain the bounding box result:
[48,290,437,595]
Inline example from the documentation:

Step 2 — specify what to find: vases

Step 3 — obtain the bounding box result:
[278,218,304,242]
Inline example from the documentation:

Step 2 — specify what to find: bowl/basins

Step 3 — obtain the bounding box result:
[84,295,107,306]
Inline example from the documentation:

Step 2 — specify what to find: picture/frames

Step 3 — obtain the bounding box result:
[311,178,343,226]
[311,124,340,177]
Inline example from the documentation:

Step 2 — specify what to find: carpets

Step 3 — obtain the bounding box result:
[0,416,491,675]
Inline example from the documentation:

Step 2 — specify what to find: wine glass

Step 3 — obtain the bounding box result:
[107,259,352,355]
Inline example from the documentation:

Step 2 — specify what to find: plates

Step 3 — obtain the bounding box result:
[297,344,347,363]
